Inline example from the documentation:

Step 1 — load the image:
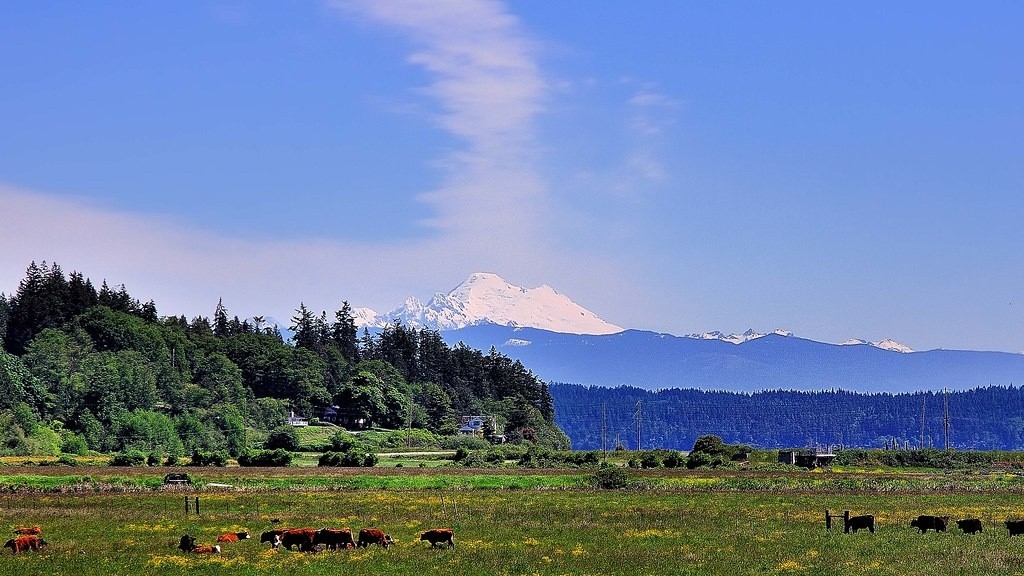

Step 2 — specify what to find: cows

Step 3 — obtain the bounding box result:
[910,515,950,535]
[261,527,356,553]
[178,534,196,554]
[3,527,48,555]
[847,515,875,534]
[358,528,388,548]
[216,532,251,543]
[193,545,222,554]
[956,519,983,535]
[1004,520,1024,538]
[420,530,454,550]
